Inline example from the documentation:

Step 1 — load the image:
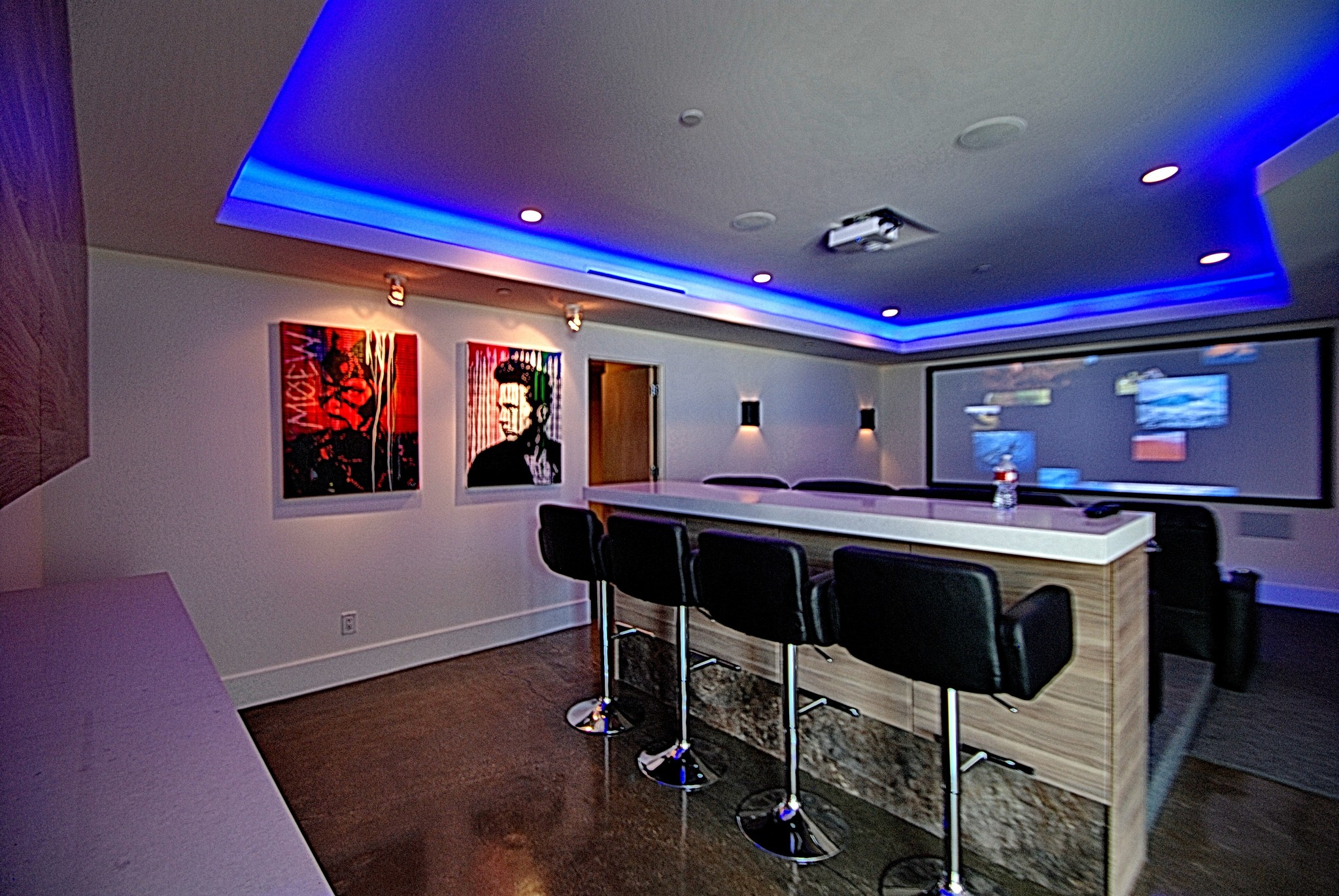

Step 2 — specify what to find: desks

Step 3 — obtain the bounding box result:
[1,574,338,895]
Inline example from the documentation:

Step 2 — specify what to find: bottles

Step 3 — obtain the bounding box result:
[991,453,1019,509]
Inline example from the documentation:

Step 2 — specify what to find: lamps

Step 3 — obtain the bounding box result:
[740,398,761,427]
[860,407,876,432]
[384,272,409,310]
[564,303,584,332]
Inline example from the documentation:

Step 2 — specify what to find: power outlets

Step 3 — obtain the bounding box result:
[343,610,358,635]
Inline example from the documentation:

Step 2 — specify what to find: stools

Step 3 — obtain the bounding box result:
[686,527,851,864]
[536,501,651,734]
[834,545,1076,895]
[601,513,730,790]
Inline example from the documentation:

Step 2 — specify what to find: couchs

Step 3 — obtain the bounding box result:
[1094,493,1259,691]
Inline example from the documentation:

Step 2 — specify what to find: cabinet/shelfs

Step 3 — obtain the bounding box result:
[2,0,93,513]
